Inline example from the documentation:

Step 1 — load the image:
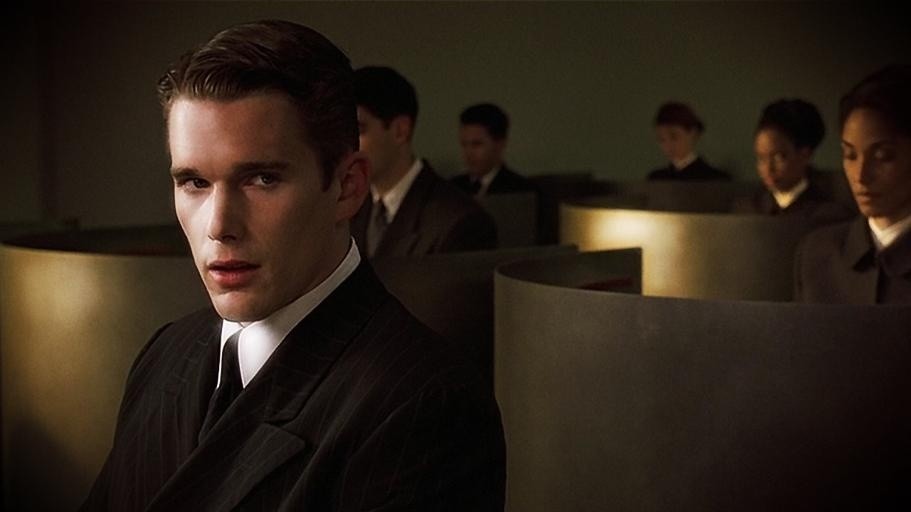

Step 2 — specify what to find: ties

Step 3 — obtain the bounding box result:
[369,199,383,228]
[470,179,482,194]
[196,326,245,443]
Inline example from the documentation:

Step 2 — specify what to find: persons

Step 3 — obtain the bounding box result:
[85,23,509,511]
[444,98,547,257]
[733,96,843,218]
[785,98,911,304]
[348,64,478,254]
[642,98,741,189]
